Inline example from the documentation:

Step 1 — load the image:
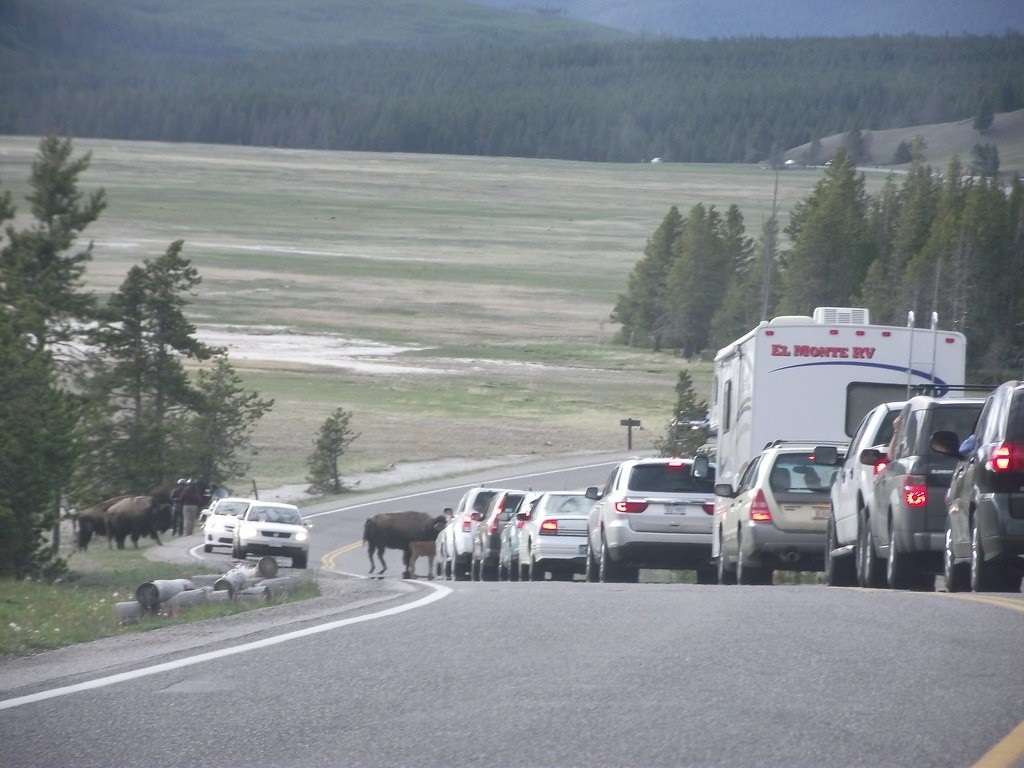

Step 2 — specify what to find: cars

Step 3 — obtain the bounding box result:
[928,380,1024,594]
[860,383,999,589]
[673,412,718,440]
[812,401,909,588]
[584,456,716,584]
[201,498,253,553]
[433,486,601,582]
[234,502,315,569]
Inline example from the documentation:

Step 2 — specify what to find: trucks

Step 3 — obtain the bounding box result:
[690,307,967,586]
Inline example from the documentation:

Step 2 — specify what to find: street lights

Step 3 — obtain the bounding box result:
[760,158,799,322]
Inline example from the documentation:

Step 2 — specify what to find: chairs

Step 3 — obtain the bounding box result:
[773,468,791,492]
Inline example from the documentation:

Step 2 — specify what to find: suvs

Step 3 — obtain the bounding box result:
[717,439,851,587]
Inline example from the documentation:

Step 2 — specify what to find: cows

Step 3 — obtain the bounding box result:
[73,494,174,550]
[361,511,450,576]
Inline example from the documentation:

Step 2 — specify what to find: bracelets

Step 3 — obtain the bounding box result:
[893,432,900,433]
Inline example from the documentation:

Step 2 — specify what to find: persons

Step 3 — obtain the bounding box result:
[169,478,202,537]
[887,416,902,461]
[958,434,976,454]
